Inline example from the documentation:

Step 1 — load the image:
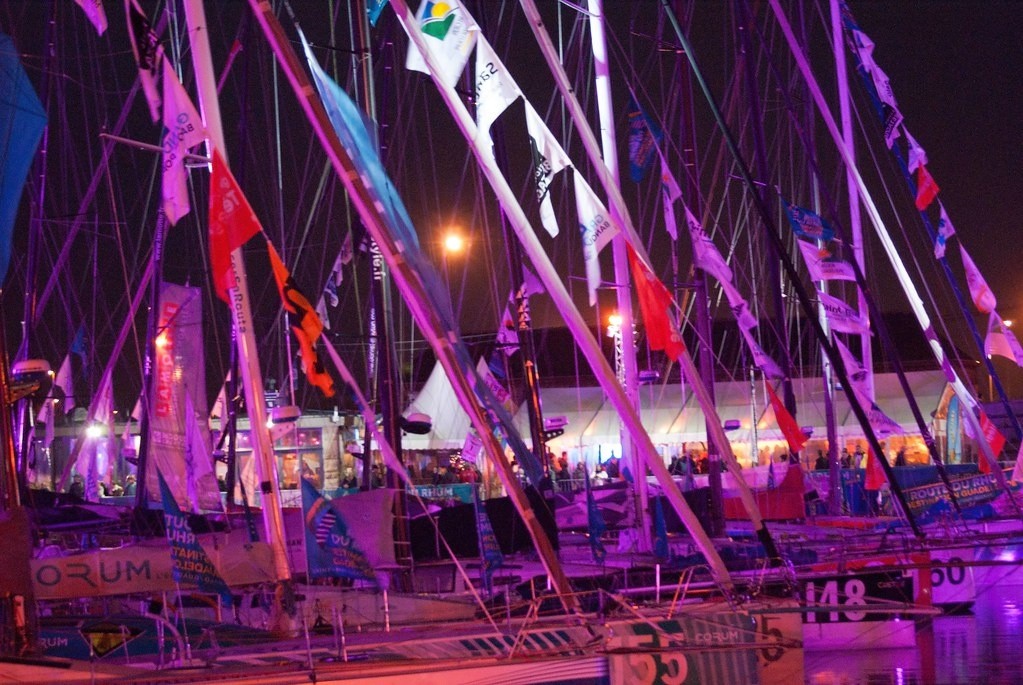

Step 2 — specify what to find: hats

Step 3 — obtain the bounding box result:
[72,473,80,478]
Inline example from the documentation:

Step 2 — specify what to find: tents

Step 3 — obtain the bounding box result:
[357,360,477,453]
[477,369,964,486]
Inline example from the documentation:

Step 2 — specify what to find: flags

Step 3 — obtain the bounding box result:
[584,463,607,564]
[156,466,234,615]
[300,475,380,595]
[471,483,504,597]
[75,0,1023,492]
[653,495,670,562]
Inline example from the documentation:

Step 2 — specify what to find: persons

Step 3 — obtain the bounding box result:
[37,442,979,499]
